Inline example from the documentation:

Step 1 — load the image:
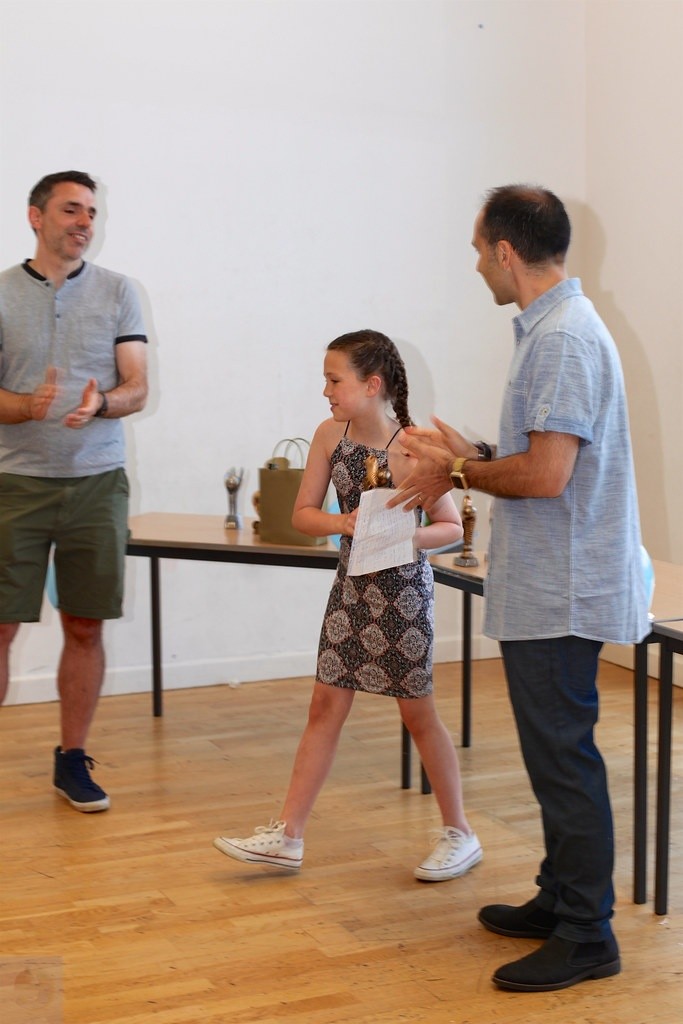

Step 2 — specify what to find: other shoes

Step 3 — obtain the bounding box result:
[491,934,621,991]
[479,899,560,939]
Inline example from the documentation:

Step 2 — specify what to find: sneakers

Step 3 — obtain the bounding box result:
[414,826,485,880]
[213,818,304,870]
[52,746,110,812]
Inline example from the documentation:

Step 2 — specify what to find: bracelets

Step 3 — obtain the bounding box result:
[475,442,491,463]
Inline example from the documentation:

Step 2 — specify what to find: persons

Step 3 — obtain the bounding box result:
[211,328,487,885]
[385,183,660,994]
[1,168,151,813]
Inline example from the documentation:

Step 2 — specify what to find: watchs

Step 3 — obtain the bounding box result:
[94,392,107,417]
[450,457,473,492]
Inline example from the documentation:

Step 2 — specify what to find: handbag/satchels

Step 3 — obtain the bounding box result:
[259,438,328,546]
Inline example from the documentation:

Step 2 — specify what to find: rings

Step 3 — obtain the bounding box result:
[418,496,423,502]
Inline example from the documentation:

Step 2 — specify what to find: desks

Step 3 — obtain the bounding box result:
[116,513,683,917]
